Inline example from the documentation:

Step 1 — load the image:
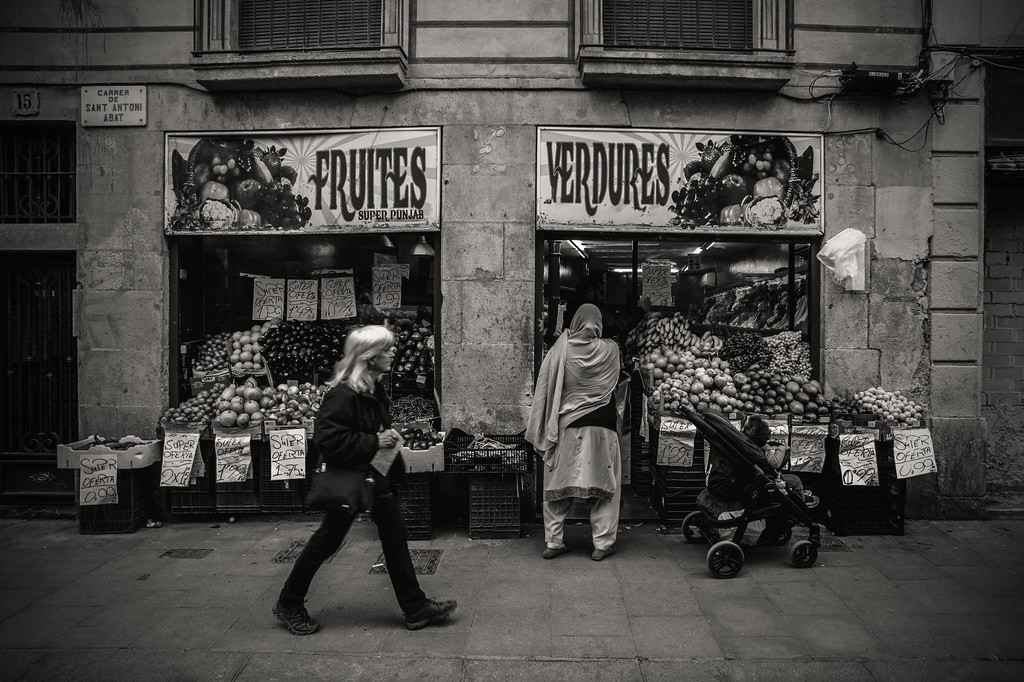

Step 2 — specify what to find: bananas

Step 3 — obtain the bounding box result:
[625,310,723,360]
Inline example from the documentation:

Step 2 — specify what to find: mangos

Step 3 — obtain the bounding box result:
[740,371,830,414]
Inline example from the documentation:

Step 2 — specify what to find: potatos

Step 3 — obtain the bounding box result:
[855,387,922,428]
[653,357,739,414]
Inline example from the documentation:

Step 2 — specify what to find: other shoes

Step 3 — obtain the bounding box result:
[591,543,616,561]
[543,543,574,559]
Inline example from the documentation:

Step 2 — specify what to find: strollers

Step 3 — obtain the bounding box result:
[677,404,828,578]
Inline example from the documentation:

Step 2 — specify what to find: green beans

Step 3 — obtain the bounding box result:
[389,395,434,423]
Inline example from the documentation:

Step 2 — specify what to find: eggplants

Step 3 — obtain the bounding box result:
[257,319,349,375]
[400,429,441,450]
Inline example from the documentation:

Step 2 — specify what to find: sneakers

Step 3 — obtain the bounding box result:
[272,588,322,636]
[804,490,820,509]
[404,596,458,631]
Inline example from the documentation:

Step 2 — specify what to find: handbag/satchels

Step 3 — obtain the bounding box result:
[614,341,633,437]
[305,468,369,518]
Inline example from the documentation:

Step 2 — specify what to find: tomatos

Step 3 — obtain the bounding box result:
[161,388,221,422]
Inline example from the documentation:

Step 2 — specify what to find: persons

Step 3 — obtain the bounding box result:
[524,304,625,559]
[271,325,458,635]
[742,416,819,508]
